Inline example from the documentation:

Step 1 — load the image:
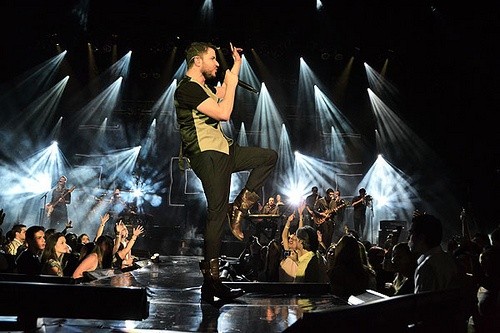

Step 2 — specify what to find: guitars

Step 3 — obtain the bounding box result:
[45,185,77,217]
[313,201,349,224]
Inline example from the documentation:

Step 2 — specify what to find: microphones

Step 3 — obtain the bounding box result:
[238,80,260,95]
[41,192,48,199]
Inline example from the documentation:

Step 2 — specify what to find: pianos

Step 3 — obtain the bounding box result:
[245,213,284,219]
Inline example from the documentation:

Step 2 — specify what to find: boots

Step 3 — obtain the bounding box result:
[199,257,244,304]
[227,189,261,242]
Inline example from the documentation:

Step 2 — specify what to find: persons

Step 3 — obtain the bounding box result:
[173,42,279,300]
[251,187,367,253]
[5,213,144,278]
[277,209,500,333]
[45,176,71,231]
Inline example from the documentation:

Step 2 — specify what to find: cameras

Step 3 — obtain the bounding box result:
[283,250,289,256]
